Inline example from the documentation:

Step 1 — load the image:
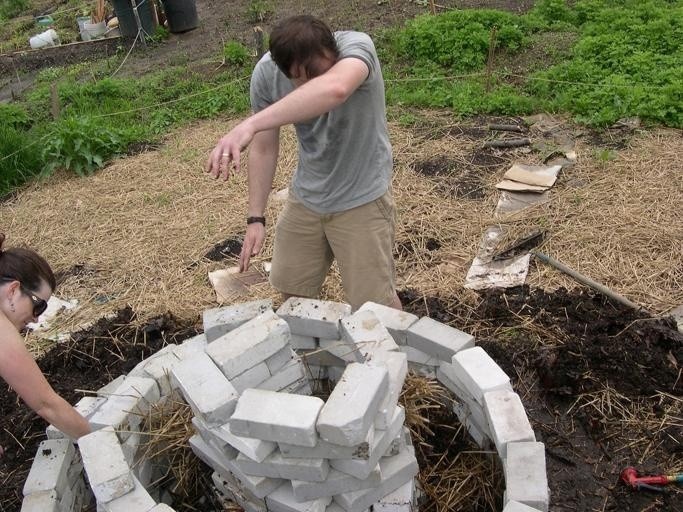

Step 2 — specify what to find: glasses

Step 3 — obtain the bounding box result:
[1,276,48,318]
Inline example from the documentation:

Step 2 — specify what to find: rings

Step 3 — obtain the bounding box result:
[222,153,230,156]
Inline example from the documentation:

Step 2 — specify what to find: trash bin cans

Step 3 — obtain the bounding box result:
[110,0,199,38]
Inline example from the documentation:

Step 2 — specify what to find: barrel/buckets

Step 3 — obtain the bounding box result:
[109,0,157,38]
[77,17,92,41]
[28,29,61,49]
[103,26,121,39]
[162,0,199,32]
[83,19,107,41]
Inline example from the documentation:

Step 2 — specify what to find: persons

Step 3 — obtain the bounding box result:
[204,16,403,308]
[0,233,89,438]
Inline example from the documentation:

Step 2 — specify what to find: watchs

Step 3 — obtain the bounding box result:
[246,217,268,225]
